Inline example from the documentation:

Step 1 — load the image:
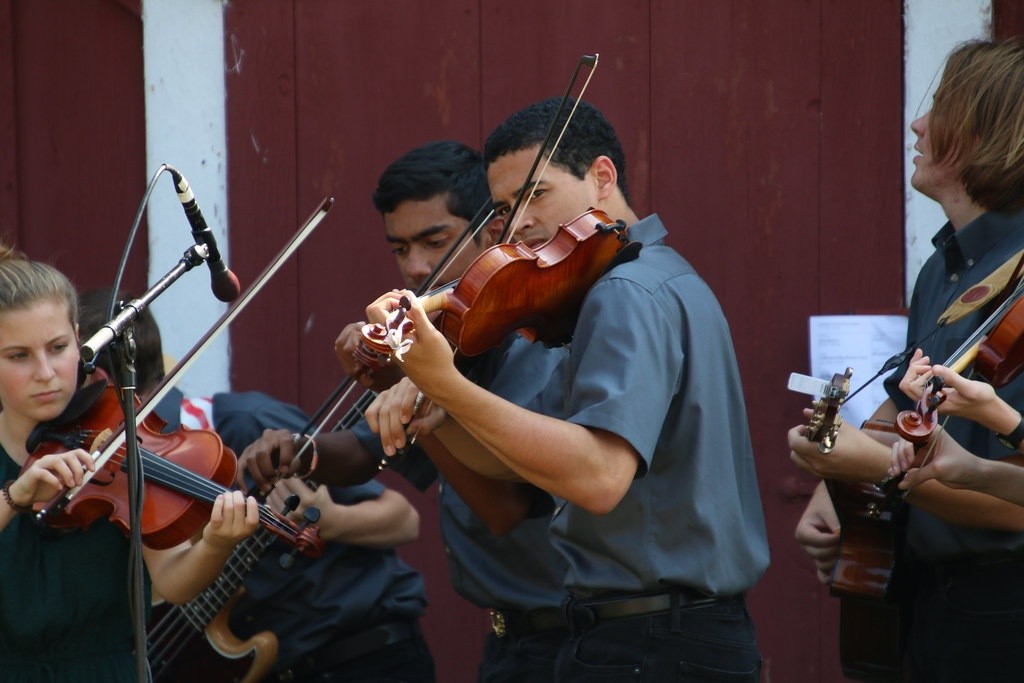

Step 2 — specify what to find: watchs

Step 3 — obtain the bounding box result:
[994,416,1024,449]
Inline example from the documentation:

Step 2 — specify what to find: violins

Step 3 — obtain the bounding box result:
[352,206,631,374]
[13,366,325,558]
[889,276,1024,442]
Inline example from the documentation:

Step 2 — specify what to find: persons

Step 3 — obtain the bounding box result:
[235,101,770,683]
[0,245,435,683]
[788,33,1024,683]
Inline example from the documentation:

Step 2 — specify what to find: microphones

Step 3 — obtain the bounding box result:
[172,170,240,302]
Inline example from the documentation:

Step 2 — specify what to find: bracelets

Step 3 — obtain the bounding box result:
[3,481,34,511]
[292,433,318,478]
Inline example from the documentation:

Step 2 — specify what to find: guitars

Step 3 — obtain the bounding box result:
[800,366,908,683]
[148,387,389,683]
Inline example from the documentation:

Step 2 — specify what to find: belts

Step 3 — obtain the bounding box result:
[490,607,565,639]
[272,618,423,683]
[562,586,722,629]
